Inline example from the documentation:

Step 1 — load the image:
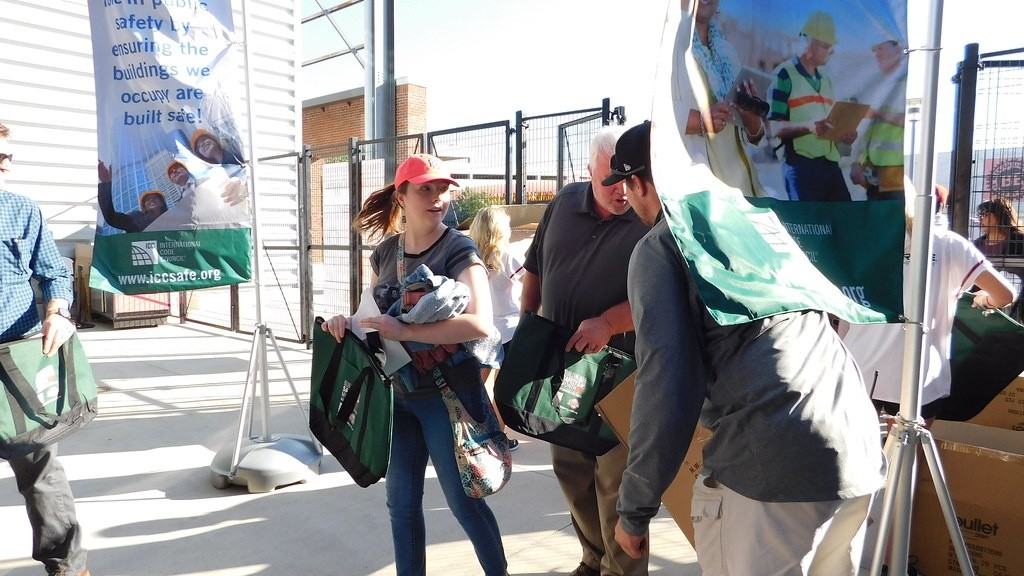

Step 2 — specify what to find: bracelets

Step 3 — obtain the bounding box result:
[700,111,705,134]
[986,295,994,307]
[745,119,762,137]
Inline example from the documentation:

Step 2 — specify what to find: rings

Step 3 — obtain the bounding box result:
[587,345,594,351]
[723,121,725,125]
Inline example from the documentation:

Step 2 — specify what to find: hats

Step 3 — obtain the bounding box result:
[935,184,948,204]
[600,119,651,186]
[395,153,460,189]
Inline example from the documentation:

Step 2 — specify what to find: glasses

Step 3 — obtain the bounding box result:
[814,40,834,55]
[977,212,995,220]
[608,154,647,176]
[0,153,13,163]
[733,91,770,119]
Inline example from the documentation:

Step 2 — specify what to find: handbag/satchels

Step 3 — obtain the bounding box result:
[439,384,512,499]
[921,291,1023,423]
[309,315,394,489]
[0,328,98,461]
[494,309,638,457]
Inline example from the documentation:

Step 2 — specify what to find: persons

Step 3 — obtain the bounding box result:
[671,0,768,201]
[466,206,527,452]
[842,24,908,201]
[602,119,889,576]
[167,160,247,208]
[320,154,512,576]
[833,172,1016,576]
[968,198,1024,327]
[518,125,651,576]
[763,11,859,200]
[190,128,247,177]
[98,158,169,233]
[0,120,98,576]
[936,185,949,230]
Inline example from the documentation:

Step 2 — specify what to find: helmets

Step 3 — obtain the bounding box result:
[191,128,213,158]
[140,191,166,210]
[166,161,187,178]
[801,12,837,46]
[872,32,899,49]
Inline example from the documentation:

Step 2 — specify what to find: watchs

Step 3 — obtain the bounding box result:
[45,308,72,319]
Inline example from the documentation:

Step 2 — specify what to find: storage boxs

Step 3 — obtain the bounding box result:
[594,367,1024,576]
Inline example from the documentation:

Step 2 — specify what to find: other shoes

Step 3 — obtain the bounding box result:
[569,562,599,576]
[508,440,518,452]
